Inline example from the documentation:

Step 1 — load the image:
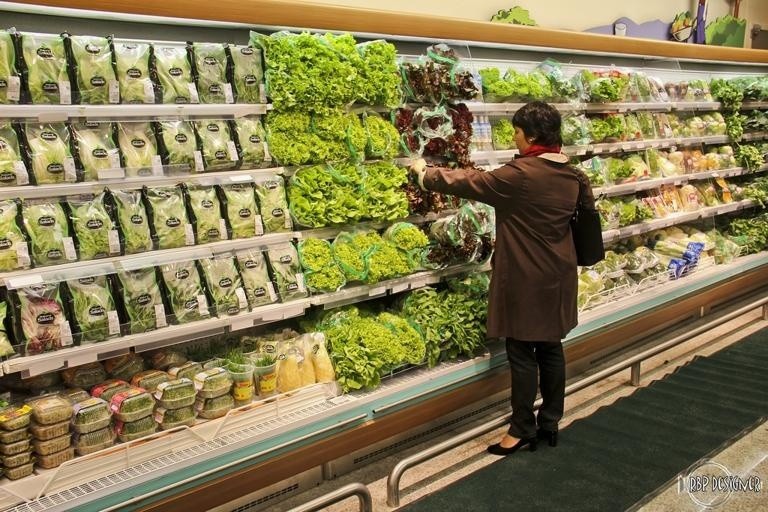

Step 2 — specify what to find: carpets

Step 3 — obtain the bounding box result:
[392,325,766,512]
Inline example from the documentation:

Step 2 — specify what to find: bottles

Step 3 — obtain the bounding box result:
[472,115,493,150]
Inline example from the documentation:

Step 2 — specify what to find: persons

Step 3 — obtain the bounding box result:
[407,100,598,457]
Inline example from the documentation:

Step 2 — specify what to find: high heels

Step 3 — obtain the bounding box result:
[488,436,538,455]
[537,426,558,447]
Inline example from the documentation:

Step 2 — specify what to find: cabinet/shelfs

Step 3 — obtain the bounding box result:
[2,1,767,512]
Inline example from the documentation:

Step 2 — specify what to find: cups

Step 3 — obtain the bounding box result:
[184,334,279,406]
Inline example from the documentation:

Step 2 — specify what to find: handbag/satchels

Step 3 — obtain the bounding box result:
[570,204,604,266]
